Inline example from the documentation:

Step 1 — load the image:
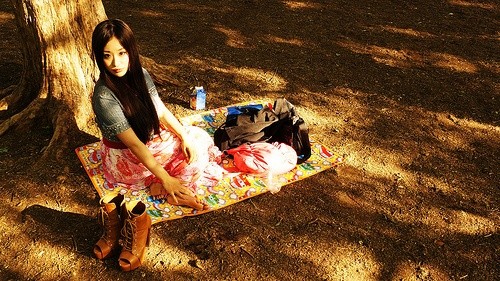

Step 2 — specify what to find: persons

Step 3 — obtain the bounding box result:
[90,17,214,213]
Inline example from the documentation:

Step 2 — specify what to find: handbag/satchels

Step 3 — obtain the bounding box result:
[215,139,298,175]
[214,92,312,165]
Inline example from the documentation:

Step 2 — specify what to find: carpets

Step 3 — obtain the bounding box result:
[73,97,347,228]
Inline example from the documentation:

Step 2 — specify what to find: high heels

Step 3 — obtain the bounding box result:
[117,199,152,272]
[93,191,129,258]
